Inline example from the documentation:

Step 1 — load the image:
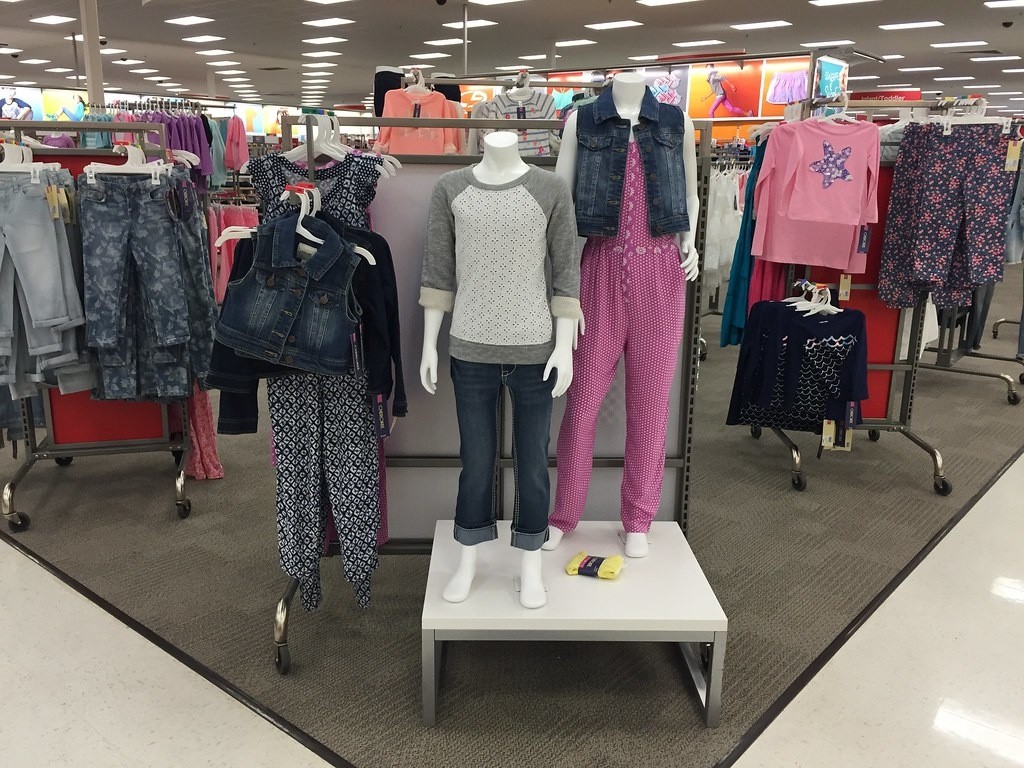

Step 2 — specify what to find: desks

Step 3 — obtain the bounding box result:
[421,518,730,730]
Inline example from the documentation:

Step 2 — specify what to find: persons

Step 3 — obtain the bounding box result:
[701,64,754,118]
[415,130,584,610]
[552,70,700,559]
[0,87,34,121]
[812,60,826,118]
[835,65,849,115]
[45,94,87,121]
[268,107,299,136]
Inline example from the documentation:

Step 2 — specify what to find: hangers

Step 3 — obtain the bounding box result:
[711,93,860,150]
[403,67,432,96]
[509,69,533,97]
[779,281,844,318]
[0,96,403,268]
[925,98,1012,135]
[711,159,753,181]
